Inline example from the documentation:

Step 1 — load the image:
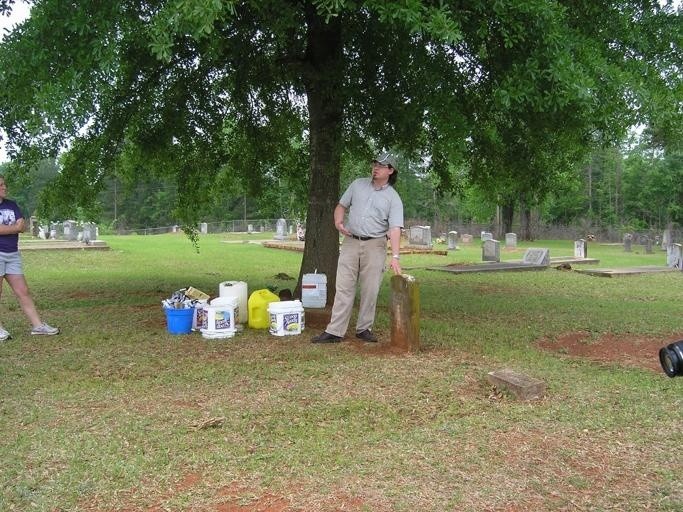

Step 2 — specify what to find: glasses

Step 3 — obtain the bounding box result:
[371,162,389,169]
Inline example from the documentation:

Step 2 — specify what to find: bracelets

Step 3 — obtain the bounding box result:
[391,254,400,260]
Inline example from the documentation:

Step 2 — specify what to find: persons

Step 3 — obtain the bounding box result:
[310,153,404,344]
[0,173,61,340]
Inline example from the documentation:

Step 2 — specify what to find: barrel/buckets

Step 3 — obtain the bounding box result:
[165,308,194,335]
[207,297,240,333]
[301,273,328,309]
[219,280,248,323]
[191,303,209,330]
[247,289,280,329]
[200,304,237,340]
[294,299,306,331]
[269,302,301,337]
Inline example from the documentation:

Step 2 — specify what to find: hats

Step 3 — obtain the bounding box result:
[373,152,398,171]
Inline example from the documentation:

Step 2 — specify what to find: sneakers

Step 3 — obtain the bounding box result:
[31,323,61,336]
[0,328,12,341]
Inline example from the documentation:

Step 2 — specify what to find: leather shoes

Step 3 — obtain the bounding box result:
[311,331,343,344]
[355,329,377,342]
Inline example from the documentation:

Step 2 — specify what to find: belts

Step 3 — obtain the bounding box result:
[349,234,375,241]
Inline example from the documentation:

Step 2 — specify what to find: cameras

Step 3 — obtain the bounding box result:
[658,340,683,378]
